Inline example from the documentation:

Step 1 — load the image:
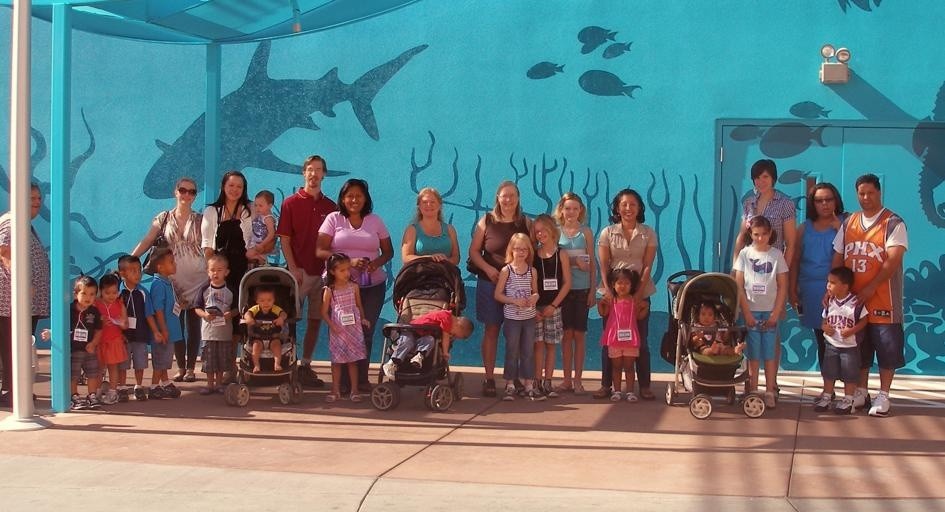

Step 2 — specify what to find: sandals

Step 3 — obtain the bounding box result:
[325,391,362,403]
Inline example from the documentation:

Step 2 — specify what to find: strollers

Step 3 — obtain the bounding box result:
[663,268,768,416]
[225,266,303,405]
[369,255,465,407]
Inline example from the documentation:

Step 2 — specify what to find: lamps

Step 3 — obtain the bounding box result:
[818,43,852,85]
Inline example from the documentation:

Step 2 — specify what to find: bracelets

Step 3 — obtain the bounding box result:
[548,304,557,309]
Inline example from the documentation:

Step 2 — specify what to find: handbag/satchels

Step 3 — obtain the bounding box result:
[661,289,687,365]
[143,211,169,275]
[466,251,488,273]
[215,206,230,253]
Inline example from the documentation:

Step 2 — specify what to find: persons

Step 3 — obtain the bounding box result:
[246,189,276,264]
[379,310,473,381]
[241,284,286,374]
[790,182,850,402]
[551,191,597,396]
[468,181,537,395]
[90,270,127,406]
[529,214,571,397]
[131,179,205,382]
[830,173,909,417]
[808,268,869,415]
[114,254,164,404]
[200,171,254,386]
[492,232,546,402]
[312,178,394,395]
[146,248,184,400]
[39,276,103,410]
[1,182,51,412]
[274,154,338,388]
[596,266,650,404]
[319,253,372,403]
[599,189,658,401]
[733,216,789,410]
[733,159,796,399]
[191,254,238,395]
[692,301,747,355]
[400,188,460,266]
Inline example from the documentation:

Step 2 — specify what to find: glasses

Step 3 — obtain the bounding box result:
[812,197,835,203]
[177,187,197,194]
[512,248,531,252]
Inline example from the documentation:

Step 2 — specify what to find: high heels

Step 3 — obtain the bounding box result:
[222,366,238,384]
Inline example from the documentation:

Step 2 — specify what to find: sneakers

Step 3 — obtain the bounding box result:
[0,390,13,408]
[383,359,396,380]
[200,387,225,394]
[764,391,776,409]
[298,366,324,387]
[71,372,195,410]
[410,352,424,370]
[814,393,891,417]
[483,378,655,402]
